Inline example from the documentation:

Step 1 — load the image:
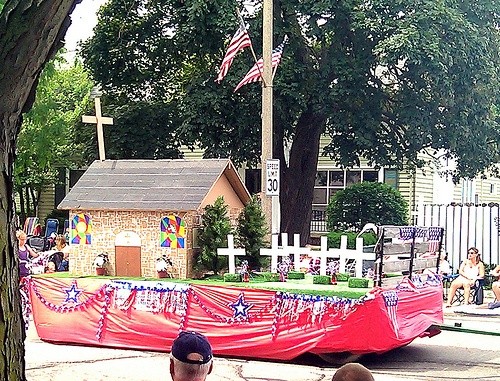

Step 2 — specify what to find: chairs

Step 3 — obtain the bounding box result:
[442,266,500,310]
[23,215,69,251]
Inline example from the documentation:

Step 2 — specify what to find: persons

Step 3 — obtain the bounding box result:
[445,247,485,309]
[332,363,375,381]
[16,230,70,280]
[487,265,500,309]
[169,331,212,381]
[422,248,450,301]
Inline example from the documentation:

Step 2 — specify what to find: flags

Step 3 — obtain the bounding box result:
[214,23,252,83]
[233,40,285,93]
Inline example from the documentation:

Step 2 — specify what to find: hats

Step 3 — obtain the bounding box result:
[172,331,212,364]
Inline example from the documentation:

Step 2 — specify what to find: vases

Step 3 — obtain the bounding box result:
[97,266,109,275]
[158,269,168,278]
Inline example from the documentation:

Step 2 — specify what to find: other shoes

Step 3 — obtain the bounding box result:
[488,302,500,308]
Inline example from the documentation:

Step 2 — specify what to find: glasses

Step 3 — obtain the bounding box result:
[467,252,474,254]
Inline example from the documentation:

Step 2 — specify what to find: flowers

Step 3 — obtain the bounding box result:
[93,255,109,267]
[155,256,172,271]
